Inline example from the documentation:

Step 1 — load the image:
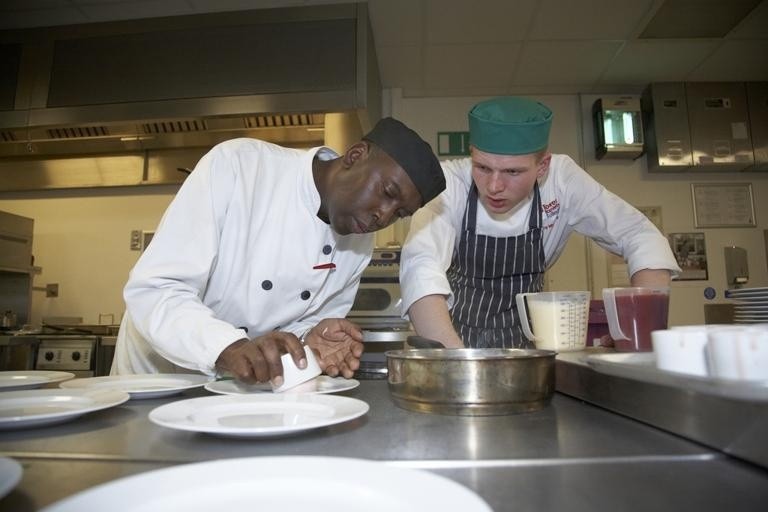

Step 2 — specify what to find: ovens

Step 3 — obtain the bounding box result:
[345,248,412,378]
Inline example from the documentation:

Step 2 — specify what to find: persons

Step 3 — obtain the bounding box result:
[396,95,684,351]
[107,116,448,387]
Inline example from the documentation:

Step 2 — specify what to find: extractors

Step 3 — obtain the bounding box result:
[0,111,315,143]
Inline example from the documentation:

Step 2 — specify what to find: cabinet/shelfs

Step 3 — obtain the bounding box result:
[641,80,766,172]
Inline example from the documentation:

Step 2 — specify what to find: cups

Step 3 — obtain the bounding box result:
[267,344,322,394]
[601,285,673,353]
[515,288,592,356]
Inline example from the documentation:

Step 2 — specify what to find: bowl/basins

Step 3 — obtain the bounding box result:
[652,324,767,384]
[383,346,558,418]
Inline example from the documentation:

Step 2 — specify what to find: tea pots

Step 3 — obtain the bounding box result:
[2,310,17,328]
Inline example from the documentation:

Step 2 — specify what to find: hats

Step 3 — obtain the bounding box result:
[468,96,553,155]
[362,117,445,207]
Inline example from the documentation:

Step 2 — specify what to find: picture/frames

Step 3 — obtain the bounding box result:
[688,181,758,229]
[604,204,665,289]
[668,231,709,282]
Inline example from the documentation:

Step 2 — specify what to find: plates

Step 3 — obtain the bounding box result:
[146,394,370,438]
[54,374,205,398]
[203,376,361,395]
[0,453,25,505]
[37,452,495,511]
[0,386,132,429]
[1,369,76,388]
[727,286,768,322]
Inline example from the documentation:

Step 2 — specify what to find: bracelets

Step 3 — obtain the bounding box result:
[298,325,312,345]
